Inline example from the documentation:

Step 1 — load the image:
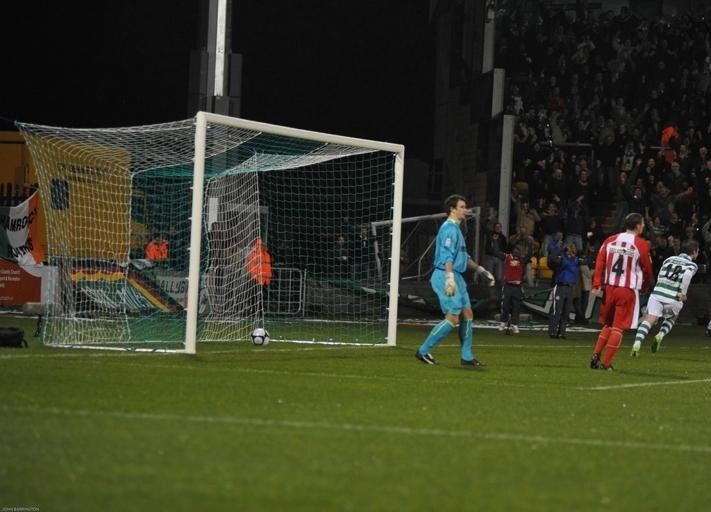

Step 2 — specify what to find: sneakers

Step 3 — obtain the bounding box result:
[590,334,660,369]
[460,358,485,368]
[416,349,437,364]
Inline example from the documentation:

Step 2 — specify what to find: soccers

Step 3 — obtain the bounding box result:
[252,329,270,346]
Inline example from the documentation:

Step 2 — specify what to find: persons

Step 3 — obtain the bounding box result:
[479,0,710,341]
[135,207,371,319]
[589,212,653,372]
[629,237,700,357]
[413,192,496,371]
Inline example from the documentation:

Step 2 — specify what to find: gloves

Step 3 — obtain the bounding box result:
[478,266,495,287]
[445,272,456,297]
